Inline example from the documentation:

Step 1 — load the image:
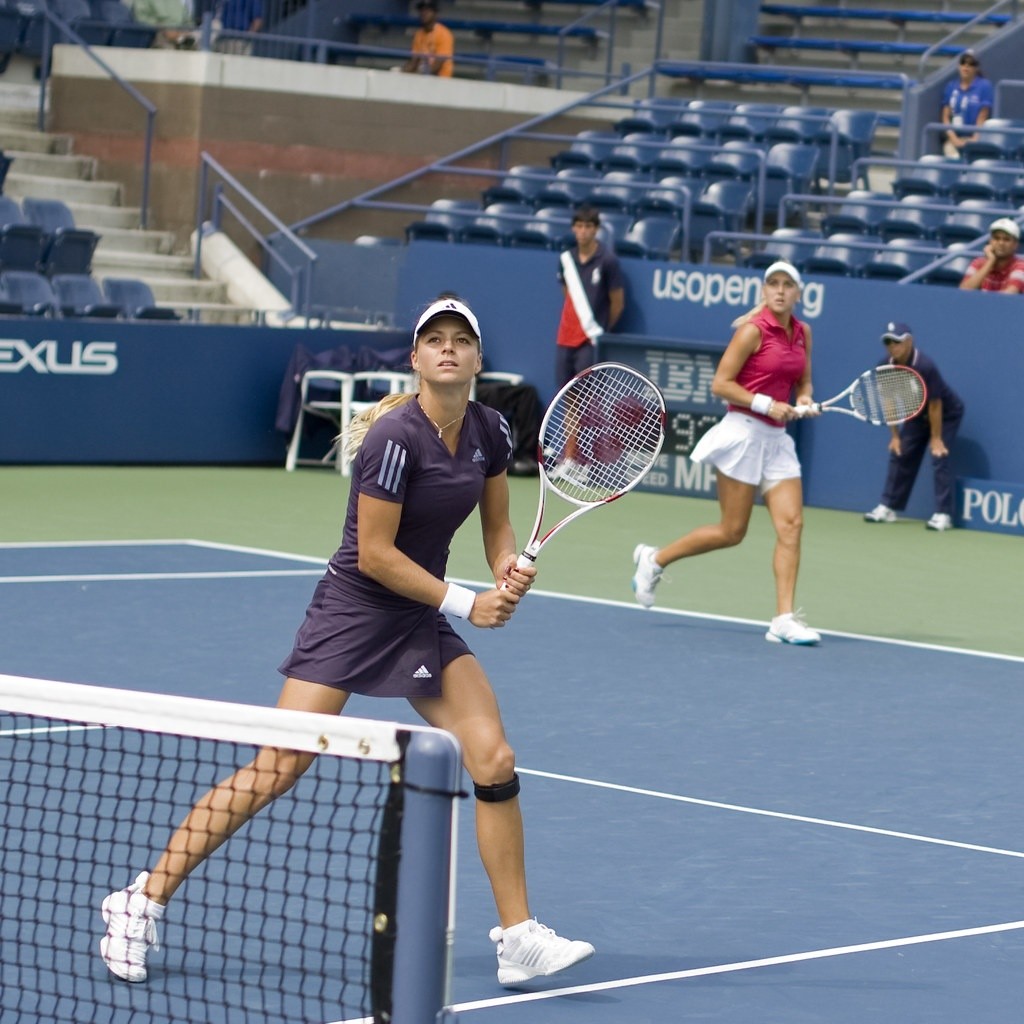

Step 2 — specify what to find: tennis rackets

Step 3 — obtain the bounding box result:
[790,364,927,427]
[498,362,667,591]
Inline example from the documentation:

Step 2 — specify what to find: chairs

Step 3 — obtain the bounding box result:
[352,77,1024,309]
[0,0,155,78]
[0,150,182,321]
[284,346,523,474]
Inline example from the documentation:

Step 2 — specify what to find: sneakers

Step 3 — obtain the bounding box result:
[99,871,160,983]
[631,544,664,607]
[545,456,588,483]
[489,915,596,988]
[864,504,897,523]
[927,512,953,531]
[764,607,821,645]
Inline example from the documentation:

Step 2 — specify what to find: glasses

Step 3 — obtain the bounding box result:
[958,59,980,66]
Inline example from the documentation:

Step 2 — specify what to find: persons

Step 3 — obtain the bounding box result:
[960,218,1024,294]
[865,322,964,530]
[213,0,262,55]
[391,1,454,78]
[633,260,820,644]
[121,0,196,49]
[547,209,623,481]
[941,54,992,158]
[100,295,594,985]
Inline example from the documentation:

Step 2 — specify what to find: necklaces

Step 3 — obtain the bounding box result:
[419,395,466,438]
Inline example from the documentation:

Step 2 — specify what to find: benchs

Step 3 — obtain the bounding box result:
[293,0,1024,87]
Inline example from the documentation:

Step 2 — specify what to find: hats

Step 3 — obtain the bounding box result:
[960,49,978,60]
[989,218,1021,240]
[413,299,481,347]
[764,262,801,289]
[880,321,912,341]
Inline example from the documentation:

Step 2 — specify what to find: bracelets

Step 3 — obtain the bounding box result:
[752,394,772,414]
[767,400,776,416]
[439,582,476,621]
